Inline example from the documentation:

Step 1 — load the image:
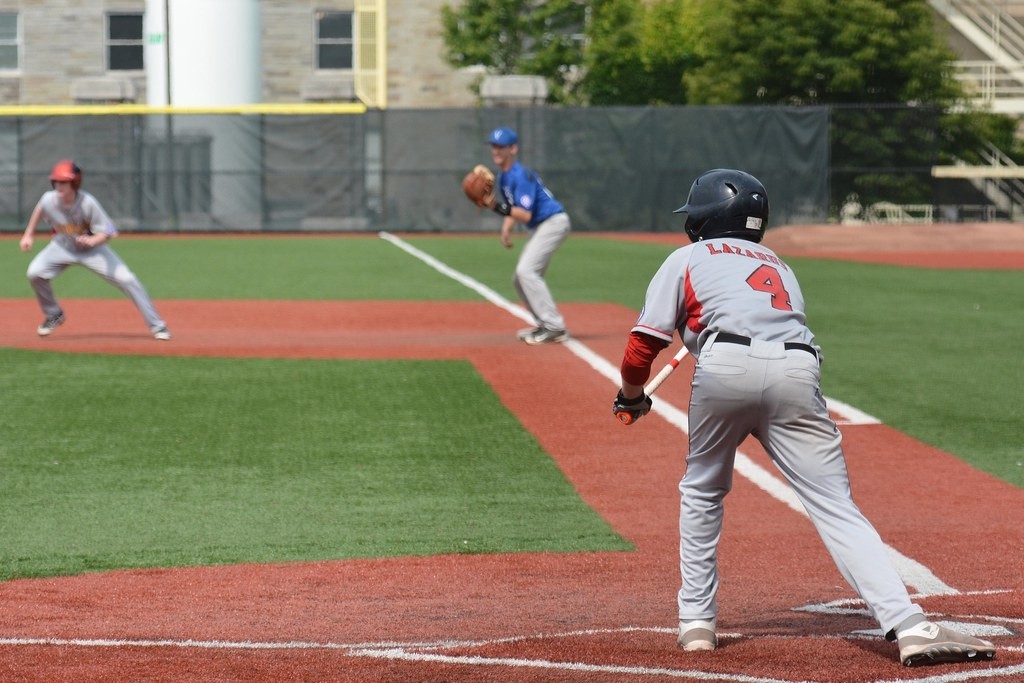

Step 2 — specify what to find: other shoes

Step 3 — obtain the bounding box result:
[154,327,171,340]
[37,311,65,335]
[516,326,569,345]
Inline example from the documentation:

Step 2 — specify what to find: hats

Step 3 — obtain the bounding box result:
[481,126,518,146]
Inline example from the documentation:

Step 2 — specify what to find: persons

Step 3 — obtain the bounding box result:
[612,167,996,666]
[20,160,170,341]
[483,127,571,345]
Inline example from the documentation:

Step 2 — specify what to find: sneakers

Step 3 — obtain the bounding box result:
[676,618,718,652]
[893,614,996,667]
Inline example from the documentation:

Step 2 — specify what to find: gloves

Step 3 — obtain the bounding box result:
[613,388,652,418]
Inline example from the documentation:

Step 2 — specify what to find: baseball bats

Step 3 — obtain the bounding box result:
[615,345,689,425]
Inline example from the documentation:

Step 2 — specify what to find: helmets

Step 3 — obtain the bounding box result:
[48,160,82,190]
[672,169,769,245]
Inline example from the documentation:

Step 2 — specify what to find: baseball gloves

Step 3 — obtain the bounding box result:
[460,163,497,210]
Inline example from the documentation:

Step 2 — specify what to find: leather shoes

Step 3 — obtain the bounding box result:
[705,332,816,360]
